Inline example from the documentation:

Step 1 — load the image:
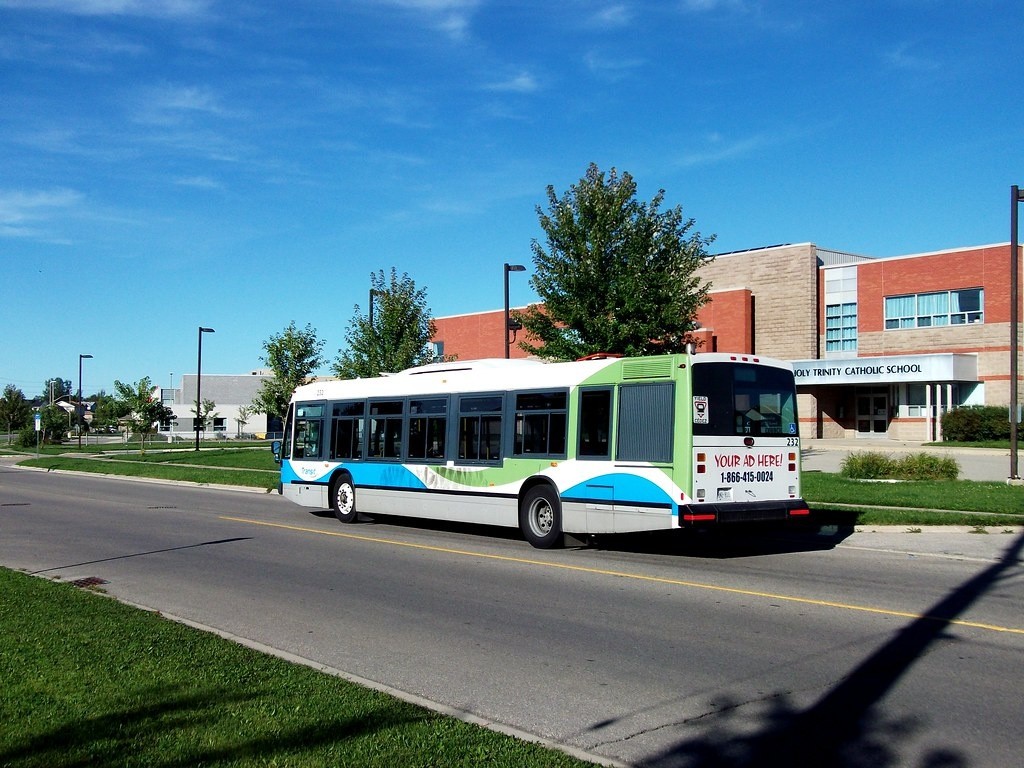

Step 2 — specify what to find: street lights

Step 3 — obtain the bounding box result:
[502,264,528,359]
[68,388,75,428]
[78,353,94,449]
[1007,184,1024,481]
[366,289,389,379]
[195,327,216,452]
[49,381,57,407]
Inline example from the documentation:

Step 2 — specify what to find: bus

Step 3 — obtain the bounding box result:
[270,338,813,550]
